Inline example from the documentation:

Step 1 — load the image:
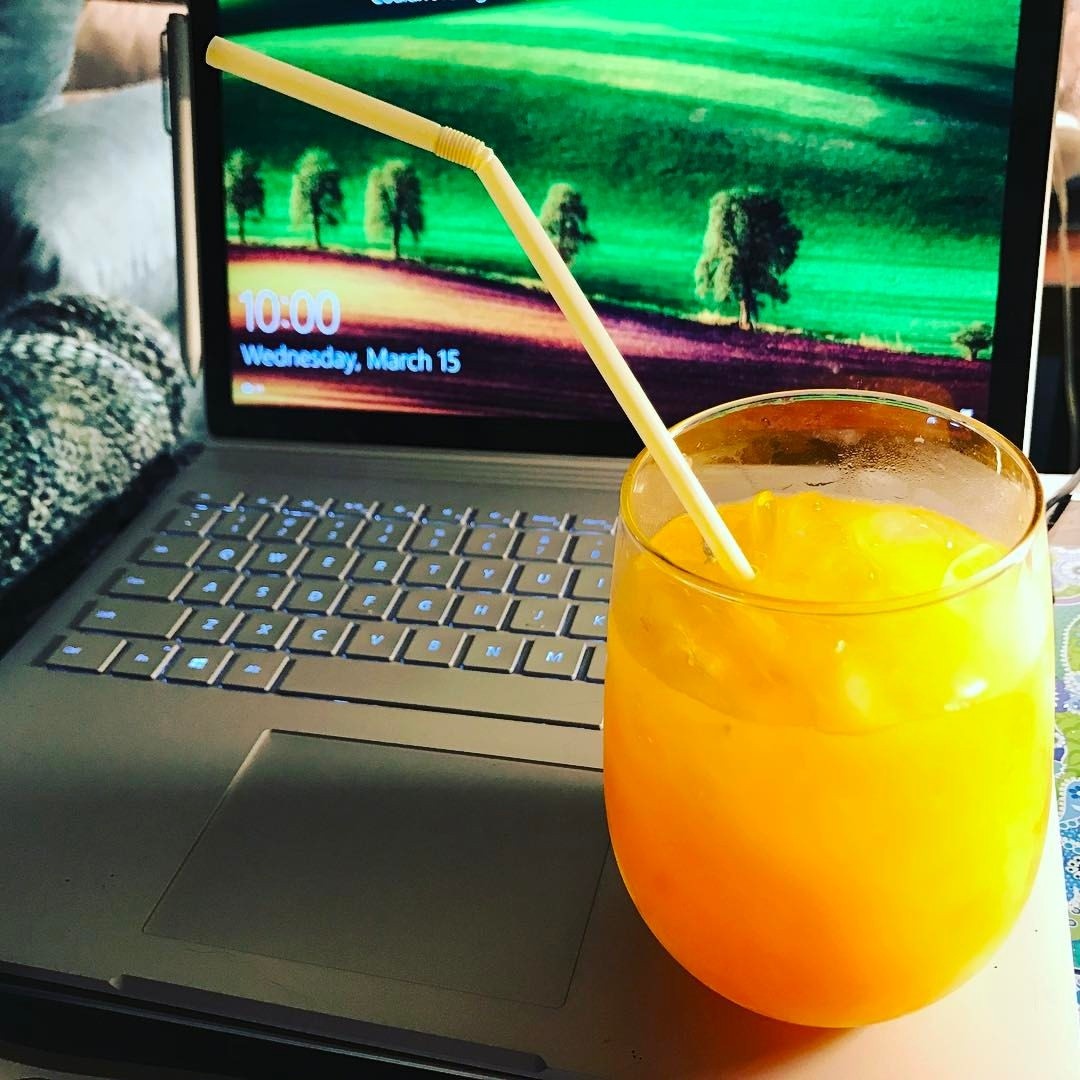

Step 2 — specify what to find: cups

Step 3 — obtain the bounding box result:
[601,387,1056,1029]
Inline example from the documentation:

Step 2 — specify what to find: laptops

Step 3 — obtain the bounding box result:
[2,1,1063,1078]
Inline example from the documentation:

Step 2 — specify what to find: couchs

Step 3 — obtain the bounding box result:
[0,0,191,321]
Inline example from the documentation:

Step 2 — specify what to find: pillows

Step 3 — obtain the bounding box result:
[0,0,84,124]
